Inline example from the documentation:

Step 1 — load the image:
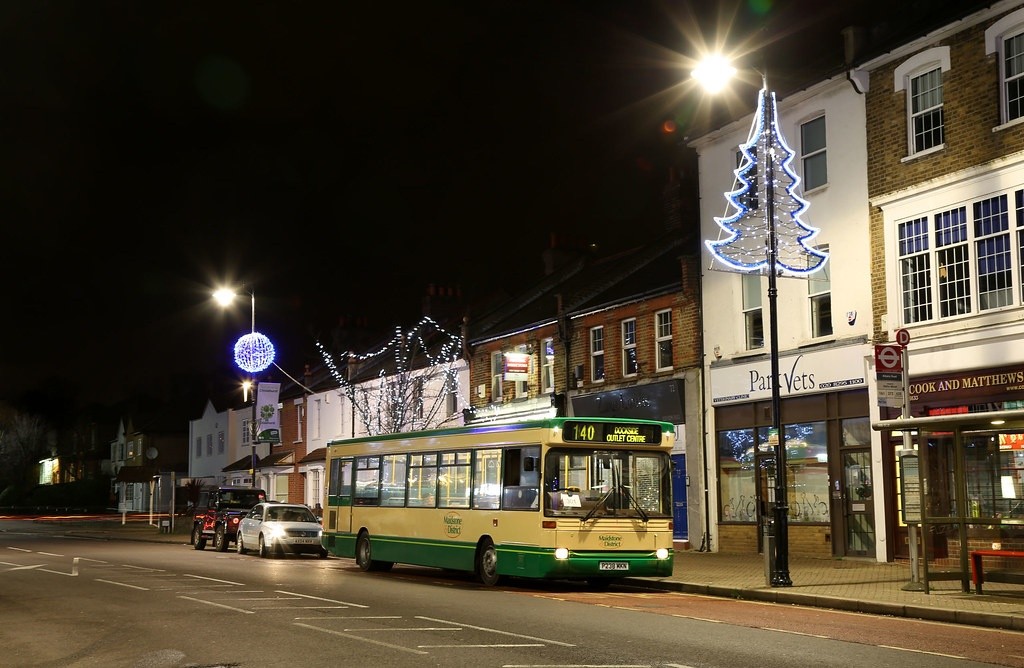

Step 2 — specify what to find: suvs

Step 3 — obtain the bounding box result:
[191,485,268,551]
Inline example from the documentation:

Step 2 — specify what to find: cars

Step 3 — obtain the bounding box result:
[236,502,329,558]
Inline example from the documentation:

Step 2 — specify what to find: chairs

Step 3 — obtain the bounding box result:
[604,492,630,511]
[547,490,587,511]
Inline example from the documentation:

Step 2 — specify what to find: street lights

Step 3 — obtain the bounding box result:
[213,287,257,488]
[692,56,793,587]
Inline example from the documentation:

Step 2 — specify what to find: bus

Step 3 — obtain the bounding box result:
[322,416,676,587]
